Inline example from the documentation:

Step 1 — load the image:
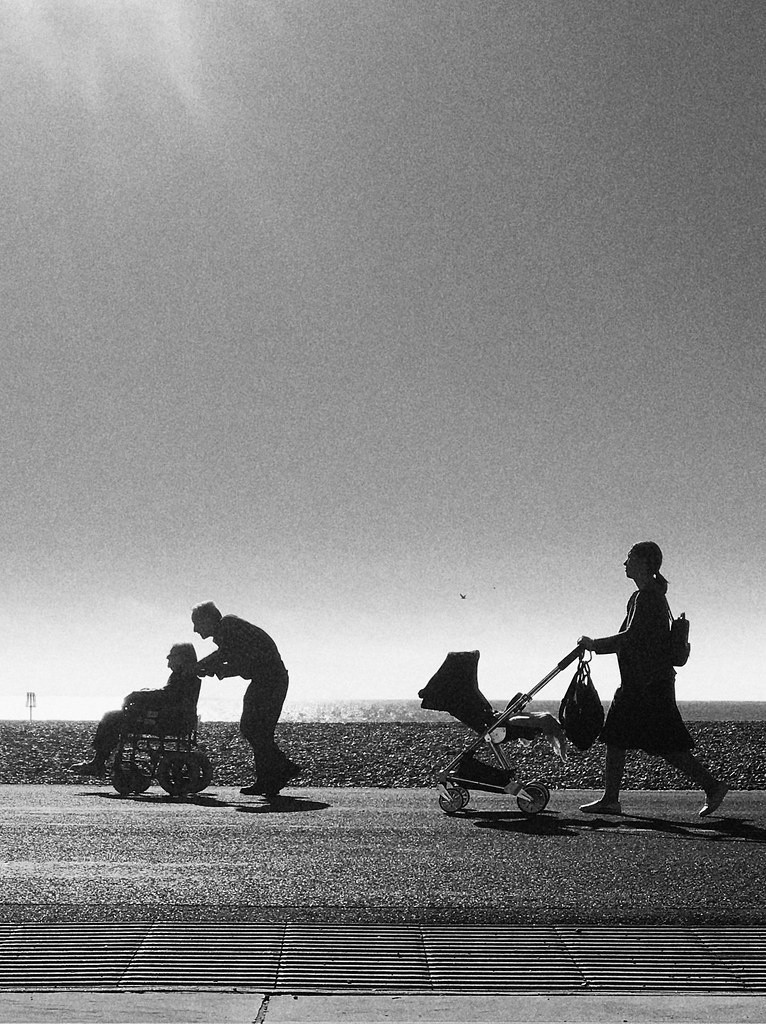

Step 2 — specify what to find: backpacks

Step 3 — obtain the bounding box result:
[636,588,690,667]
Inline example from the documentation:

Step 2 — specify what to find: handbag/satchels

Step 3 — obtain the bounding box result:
[559,661,605,751]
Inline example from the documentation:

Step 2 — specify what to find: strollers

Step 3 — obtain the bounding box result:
[416,636,589,816]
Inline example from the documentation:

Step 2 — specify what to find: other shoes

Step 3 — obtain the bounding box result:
[240,780,279,795]
[579,800,622,814]
[699,781,729,816]
[70,761,106,776]
[267,763,301,796]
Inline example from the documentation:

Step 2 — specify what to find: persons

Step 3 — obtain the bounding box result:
[69,600,300,797]
[577,541,729,816]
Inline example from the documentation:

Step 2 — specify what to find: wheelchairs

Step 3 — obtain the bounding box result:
[107,667,214,796]
